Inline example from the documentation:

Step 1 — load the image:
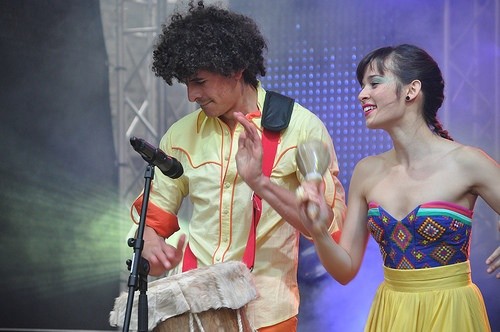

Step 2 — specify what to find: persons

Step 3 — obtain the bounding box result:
[294,44,500,332]
[131,1,346,332]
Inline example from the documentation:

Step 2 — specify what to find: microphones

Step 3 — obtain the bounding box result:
[130,137,184,180]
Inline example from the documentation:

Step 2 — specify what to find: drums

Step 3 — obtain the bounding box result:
[109,259,261,332]
